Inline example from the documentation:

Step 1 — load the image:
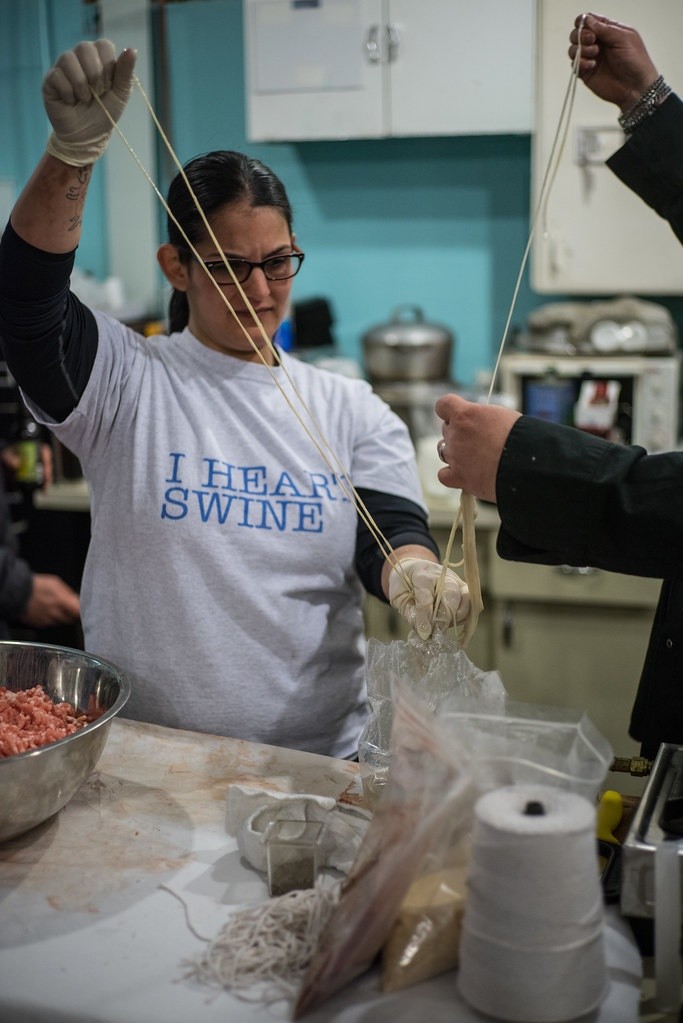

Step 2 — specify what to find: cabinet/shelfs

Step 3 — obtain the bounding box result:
[530,2,683,294]
[241,1,536,142]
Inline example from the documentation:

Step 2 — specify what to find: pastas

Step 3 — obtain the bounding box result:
[86,15,586,647]
[158,880,336,1007]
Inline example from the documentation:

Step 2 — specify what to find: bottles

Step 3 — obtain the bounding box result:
[18,399,46,485]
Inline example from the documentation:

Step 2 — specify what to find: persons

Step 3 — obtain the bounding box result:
[1,38,483,764]
[435,9,683,844]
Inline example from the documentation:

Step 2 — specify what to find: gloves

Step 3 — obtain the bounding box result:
[388,557,484,641]
[41,39,137,168]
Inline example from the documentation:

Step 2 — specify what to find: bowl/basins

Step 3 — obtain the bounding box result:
[0,641,132,842]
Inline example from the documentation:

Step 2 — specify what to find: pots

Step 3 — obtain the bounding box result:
[359,304,453,382]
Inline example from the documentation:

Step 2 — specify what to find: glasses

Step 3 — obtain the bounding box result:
[179,242,305,284]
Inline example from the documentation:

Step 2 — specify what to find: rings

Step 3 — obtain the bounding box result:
[438,441,447,464]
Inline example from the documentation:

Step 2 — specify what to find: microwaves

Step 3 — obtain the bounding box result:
[497,349,680,455]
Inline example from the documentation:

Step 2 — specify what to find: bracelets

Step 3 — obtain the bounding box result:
[618,75,671,134]
